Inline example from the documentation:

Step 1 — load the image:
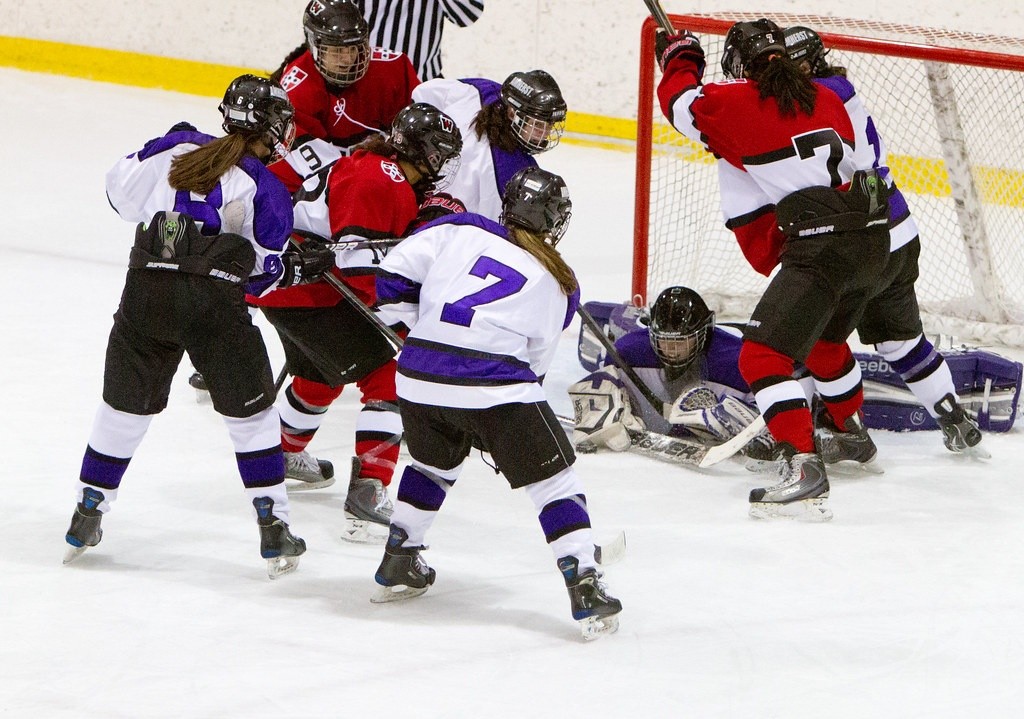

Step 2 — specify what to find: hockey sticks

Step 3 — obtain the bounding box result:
[555,412,766,469]
[323,236,407,251]
[577,303,673,421]
[289,237,405,350]
[644,0,677,39]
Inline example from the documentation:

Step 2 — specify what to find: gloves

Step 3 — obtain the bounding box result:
[268,238,337,288]
[655,26,706,79]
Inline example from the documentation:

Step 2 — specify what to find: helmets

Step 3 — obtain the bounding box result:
[387,102,463,199]
[303,0,371,89]
[782,27,827,69]
[501,70,567,154]
[720,18,785,82]
[218,75,296,168]
[647,286,715,368]
[501,167,572,248]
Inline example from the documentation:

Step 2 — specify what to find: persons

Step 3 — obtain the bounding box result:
[63,73,307,580]
[351,0,484,82]
[246,102,463,550]
[740,26,991,476]
[655,19,891,522]
[411,71,568,452]
[188,0,421,391]
[567,287,1024,454]
[370,166,623,641]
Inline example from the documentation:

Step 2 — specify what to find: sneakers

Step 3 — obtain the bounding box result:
[191,370,209,405]
[370,523,436,604]
[558,545,622,641]
[815,409,885,475]
[341,456,428,552]
[747,435,831,520]
[282,446,336,493]
[62,486,105,565]
[252,496,306,580]
[933,394,992,461]
